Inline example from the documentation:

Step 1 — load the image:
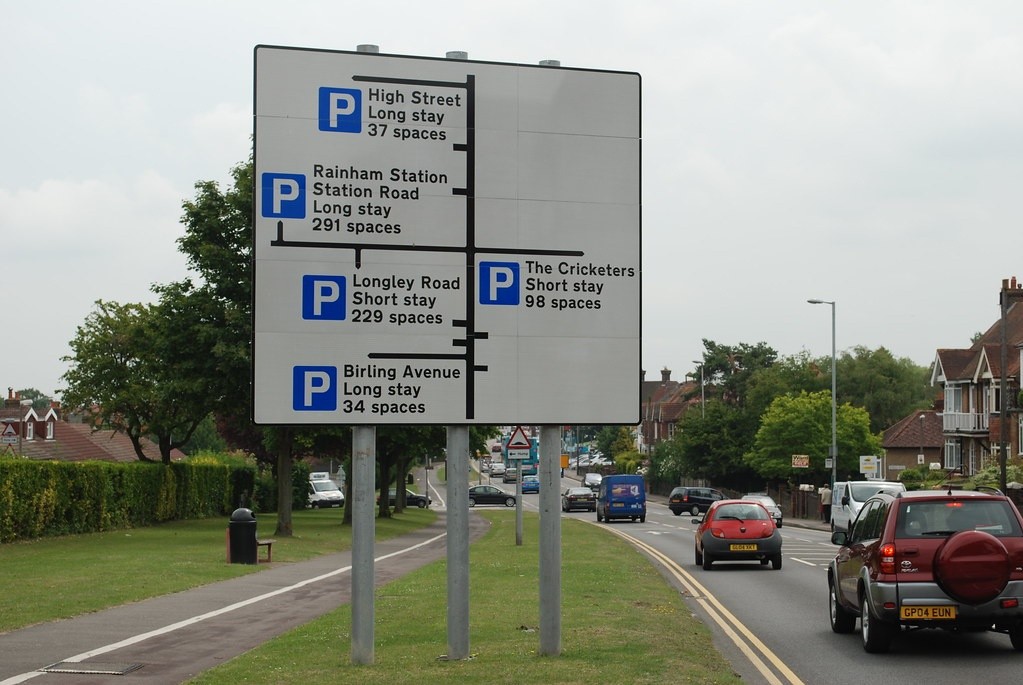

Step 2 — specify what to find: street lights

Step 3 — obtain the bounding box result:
[807,299,838,488]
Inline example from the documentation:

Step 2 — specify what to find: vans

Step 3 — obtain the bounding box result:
[593,473,646,524]
[828,480,908,541]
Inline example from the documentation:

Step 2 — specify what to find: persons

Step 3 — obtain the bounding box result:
[946,510,976,529]
[821,483,831,523]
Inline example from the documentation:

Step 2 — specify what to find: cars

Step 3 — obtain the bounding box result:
[667,486,732,516]
[469,484,516,507]
[474,442,506,478]
[307,480,345,508]
[521,475,540,494]
[502,467,517,483]
[376,487,433,509]
[568,448,616,471]
[580,472,602,491]
[740,492,783,530]
[561,487,597,513]
[690,498,784,571]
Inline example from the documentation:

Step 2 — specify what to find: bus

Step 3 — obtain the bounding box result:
[502,435,539,475]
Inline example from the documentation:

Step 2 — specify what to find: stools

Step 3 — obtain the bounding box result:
[257,538,277,562]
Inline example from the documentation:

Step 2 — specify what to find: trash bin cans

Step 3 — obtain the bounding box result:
[227,507,258,566]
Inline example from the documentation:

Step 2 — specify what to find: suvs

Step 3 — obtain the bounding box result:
[825,484,1023,661]
